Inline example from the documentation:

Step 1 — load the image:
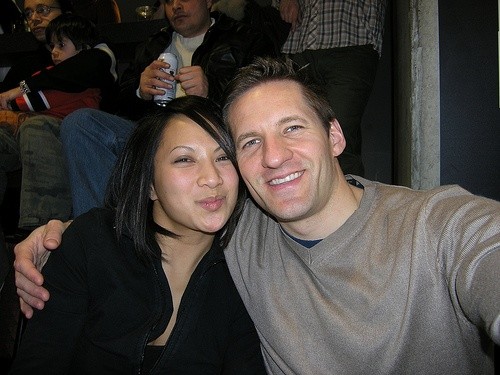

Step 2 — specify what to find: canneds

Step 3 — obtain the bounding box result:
[135,5,154,21]
[154,53,178,108]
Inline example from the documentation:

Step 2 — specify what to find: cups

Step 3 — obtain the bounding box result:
[136,3,153,20]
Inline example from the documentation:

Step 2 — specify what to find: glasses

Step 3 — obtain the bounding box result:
[24,6,60,18]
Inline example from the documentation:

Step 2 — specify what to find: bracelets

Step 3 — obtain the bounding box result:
[19,80,29,94]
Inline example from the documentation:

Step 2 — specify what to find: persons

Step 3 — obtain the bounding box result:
[0,0,118,231]
[1,12,100,130]
[273,0,386,178]
[59,0,271,220]
[12,94,269,375]
[14,58,500,375]
